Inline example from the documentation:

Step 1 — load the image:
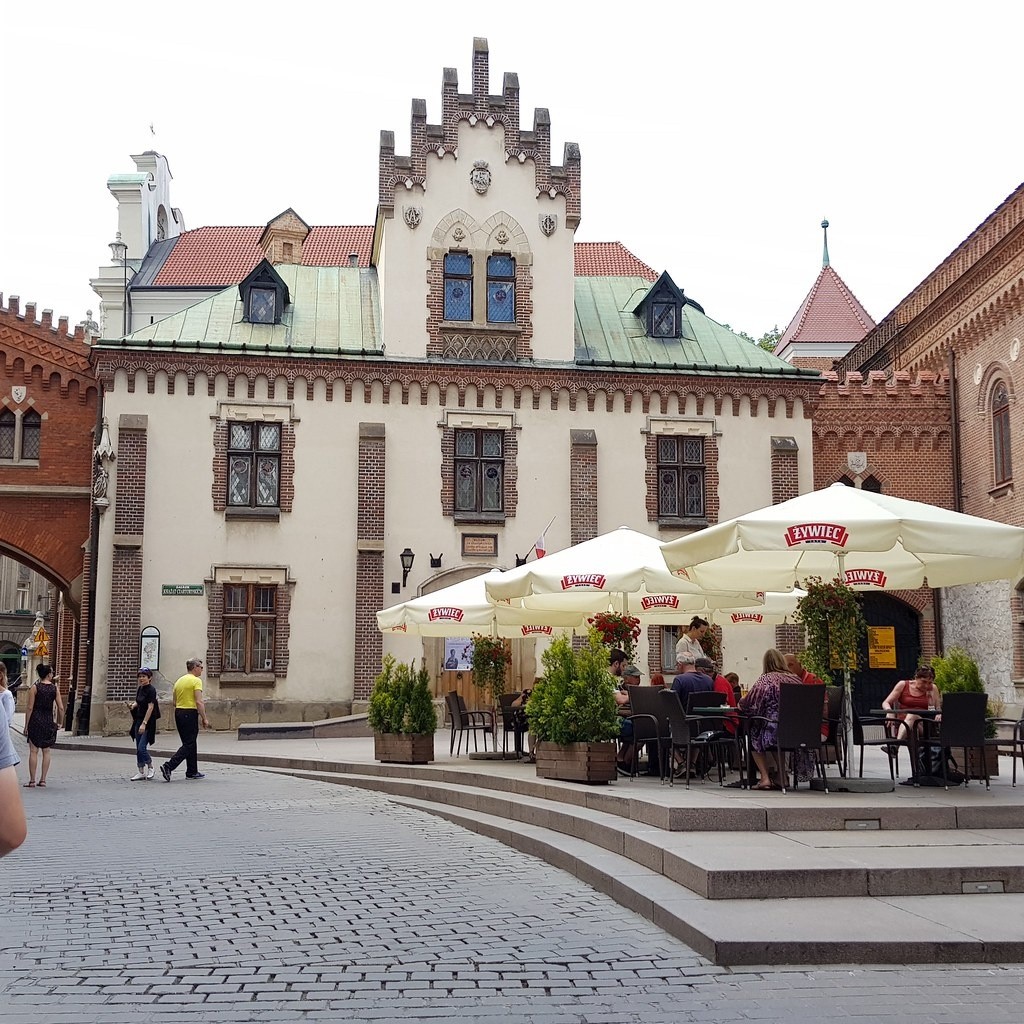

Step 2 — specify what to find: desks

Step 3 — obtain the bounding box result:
[694,707,781,790]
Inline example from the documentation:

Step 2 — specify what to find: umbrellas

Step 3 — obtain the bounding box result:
[486,525,766,663]
[375,568,590,755]
[659,482,1024,794]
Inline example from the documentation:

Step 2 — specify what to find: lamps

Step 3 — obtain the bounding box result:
[400,548,415,587]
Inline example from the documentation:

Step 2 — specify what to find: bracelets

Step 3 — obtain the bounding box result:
[142,720,147,724]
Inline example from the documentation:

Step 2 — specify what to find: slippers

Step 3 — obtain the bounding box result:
[752,778,773,789]
[771,777,790,791]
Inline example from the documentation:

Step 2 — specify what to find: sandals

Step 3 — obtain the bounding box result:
[23,781,36,787]
[36,780,46,787]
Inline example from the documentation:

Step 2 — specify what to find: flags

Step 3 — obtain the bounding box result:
[535,535,546,559]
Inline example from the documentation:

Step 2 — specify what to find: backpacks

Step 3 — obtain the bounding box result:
[920,745,951,777]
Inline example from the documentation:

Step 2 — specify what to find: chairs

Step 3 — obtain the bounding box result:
[871,709,960,786]
[446,690,527,761]
[612,683,1024,795]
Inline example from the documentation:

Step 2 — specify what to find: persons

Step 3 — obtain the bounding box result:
[880,664,942,786]
[0,661,28,859]
[724,672,742,702]
[694,655,740,776]
[127,667,156,780]
[651,673,668,687]
[661,650,715,777]
[605,647,644,776]
[23,664,65,786]
[737,649,804,789]
[615,664,645,759]
[446,649,458,669]
[160,656,209,781]
[511,677,542,763]
[712,660,718,671]
[770,653,828,788]
[676,616,716,663]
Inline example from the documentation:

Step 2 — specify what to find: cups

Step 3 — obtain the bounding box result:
[740,683,748,697]
[927,691,935,711]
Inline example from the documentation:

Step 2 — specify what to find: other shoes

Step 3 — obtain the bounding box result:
[881,745,898,758]
[528,752,536,762]
[672,762,687,778]
[147,767,154,779]
[689,764,698,779]
[131,772,147,781]
[613,757,640,776]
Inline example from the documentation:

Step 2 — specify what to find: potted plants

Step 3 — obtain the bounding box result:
[523,630,625,786]
[915,646,999,780]
[367,652,439,763]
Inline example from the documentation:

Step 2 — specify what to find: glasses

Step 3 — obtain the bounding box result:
[673,662,678,668]
[139,668,150,672]
[49,671,55,674]
[197,665,203,669]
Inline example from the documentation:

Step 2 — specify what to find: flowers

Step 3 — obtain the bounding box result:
[790,576,878,702]
[461,631,513,715]
[586,610,643,665]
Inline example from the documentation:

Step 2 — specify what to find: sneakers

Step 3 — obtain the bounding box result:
[185,770,206,780]
[160,764,171,781]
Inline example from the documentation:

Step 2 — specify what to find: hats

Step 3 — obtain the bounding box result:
[137,668,153,682]
[694,658,714,668]
[523,689,533,696]
[622,665,646,677]
